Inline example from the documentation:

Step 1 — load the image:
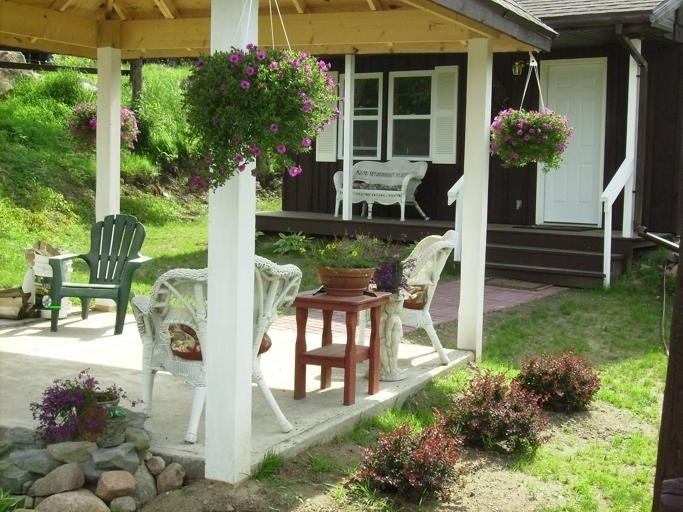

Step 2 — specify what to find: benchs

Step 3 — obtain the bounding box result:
[331,157,431,223]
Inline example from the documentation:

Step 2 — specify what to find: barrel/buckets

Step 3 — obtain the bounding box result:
[34,252,74,319]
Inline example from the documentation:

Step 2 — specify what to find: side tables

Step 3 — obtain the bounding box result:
[294,288,390,406]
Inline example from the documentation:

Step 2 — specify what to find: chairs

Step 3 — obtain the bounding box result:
[48,215,146,334]
[354,230,459,366]
[129,254,302,444]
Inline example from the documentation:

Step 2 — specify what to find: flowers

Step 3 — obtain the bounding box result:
[489,103,573,175]
[312,233,417,297]
[67,103,141,156]
[175,42,347,199]
[28,368,145,442]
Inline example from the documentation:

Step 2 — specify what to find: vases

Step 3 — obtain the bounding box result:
[73,393,118,441]
[315,262,374,297]
